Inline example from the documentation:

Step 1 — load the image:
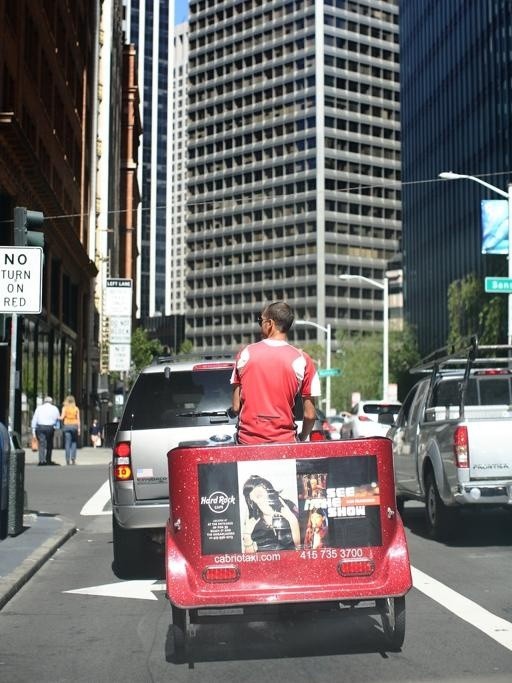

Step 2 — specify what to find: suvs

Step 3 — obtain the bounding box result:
[111,350,326,580]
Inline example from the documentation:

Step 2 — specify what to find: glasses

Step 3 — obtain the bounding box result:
[257,312,267,327]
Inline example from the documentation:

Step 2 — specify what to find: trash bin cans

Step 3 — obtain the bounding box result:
[8,430,25,537]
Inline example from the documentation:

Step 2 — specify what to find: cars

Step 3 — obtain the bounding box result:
[324,399,403,444]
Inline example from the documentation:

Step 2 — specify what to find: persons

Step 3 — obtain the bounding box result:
[228,302,321,443]
[57,396,81,465]
[31,397,60,466]
[91,419,102,448]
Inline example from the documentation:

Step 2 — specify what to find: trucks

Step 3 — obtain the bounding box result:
[378,362,512,538]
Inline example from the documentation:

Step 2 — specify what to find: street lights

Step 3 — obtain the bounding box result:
[339,275,390,399]
[290,319,334,417]
[438,171,511,358]
